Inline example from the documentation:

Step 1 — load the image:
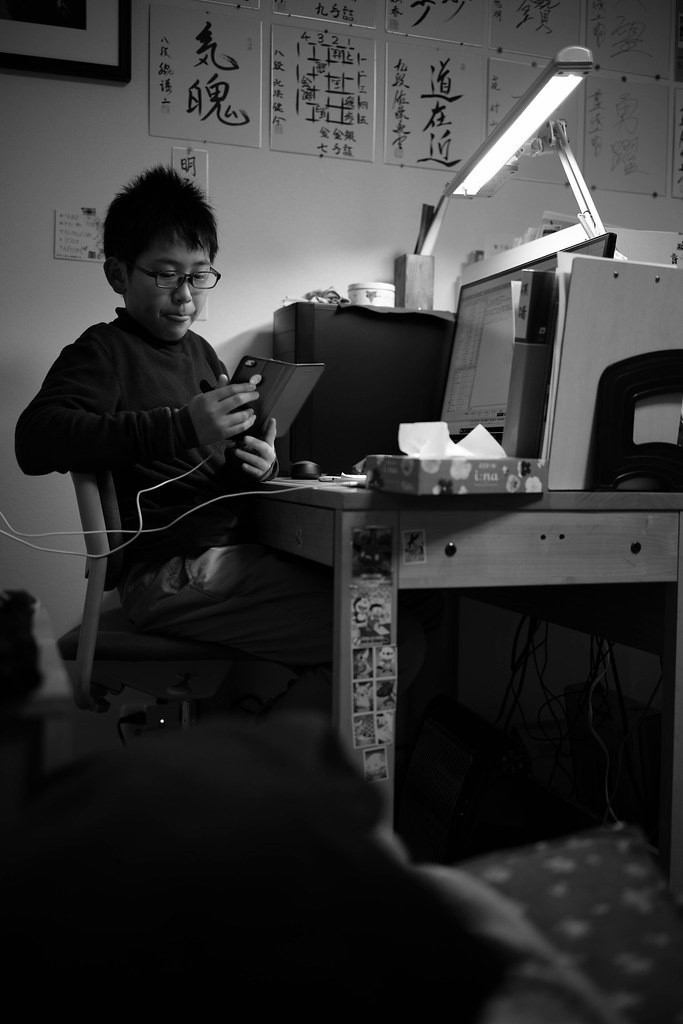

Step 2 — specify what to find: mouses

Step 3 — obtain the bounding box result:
[289,461,321,479]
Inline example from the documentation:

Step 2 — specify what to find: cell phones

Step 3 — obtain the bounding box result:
[227,353,288,446]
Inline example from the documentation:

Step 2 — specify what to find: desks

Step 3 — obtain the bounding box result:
[262,474,683,913]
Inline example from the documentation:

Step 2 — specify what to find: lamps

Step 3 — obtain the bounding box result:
[444,44,627,262]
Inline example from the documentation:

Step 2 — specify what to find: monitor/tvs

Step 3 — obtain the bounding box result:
[440,231,616,440]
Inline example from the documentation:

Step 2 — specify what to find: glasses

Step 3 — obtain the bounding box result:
[118,258,221,290]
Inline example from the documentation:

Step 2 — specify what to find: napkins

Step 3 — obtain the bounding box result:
[366,419,543,495]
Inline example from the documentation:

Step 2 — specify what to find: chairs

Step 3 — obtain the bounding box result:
[69,468,269,733]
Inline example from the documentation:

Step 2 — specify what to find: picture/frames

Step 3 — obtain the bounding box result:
[0,0,134,87]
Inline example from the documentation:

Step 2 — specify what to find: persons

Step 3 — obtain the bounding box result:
[14,165,333,669]
